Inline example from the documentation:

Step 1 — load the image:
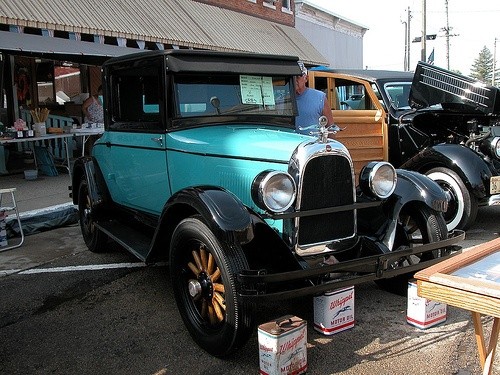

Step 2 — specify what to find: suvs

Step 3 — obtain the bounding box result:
[303,61,500,239]
[67,48,465,359]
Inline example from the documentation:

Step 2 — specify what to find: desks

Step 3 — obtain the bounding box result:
[0,126,104,181]
[412,237,500,375]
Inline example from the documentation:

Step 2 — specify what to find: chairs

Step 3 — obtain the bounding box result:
[139,95,166,118]
[0,188,25,252]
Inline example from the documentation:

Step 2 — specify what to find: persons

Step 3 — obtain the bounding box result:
[83,85,104,152]
[294,61,334,137]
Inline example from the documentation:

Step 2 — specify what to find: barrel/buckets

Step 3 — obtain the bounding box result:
[257,314,308,375]
[407,278,447,329]
[312,285,354,335]
